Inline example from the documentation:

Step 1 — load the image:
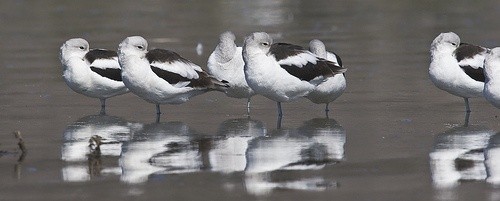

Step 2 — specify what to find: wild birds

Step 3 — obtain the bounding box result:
[59,37,133,106]
[482,46,500,109]
[428,112,500,191]
[428,32,491,112]
[241,32,348,118]
[301,39,347,110]
[207,30,258,107]
[117,36,231,115]
[59,106,349,177]
